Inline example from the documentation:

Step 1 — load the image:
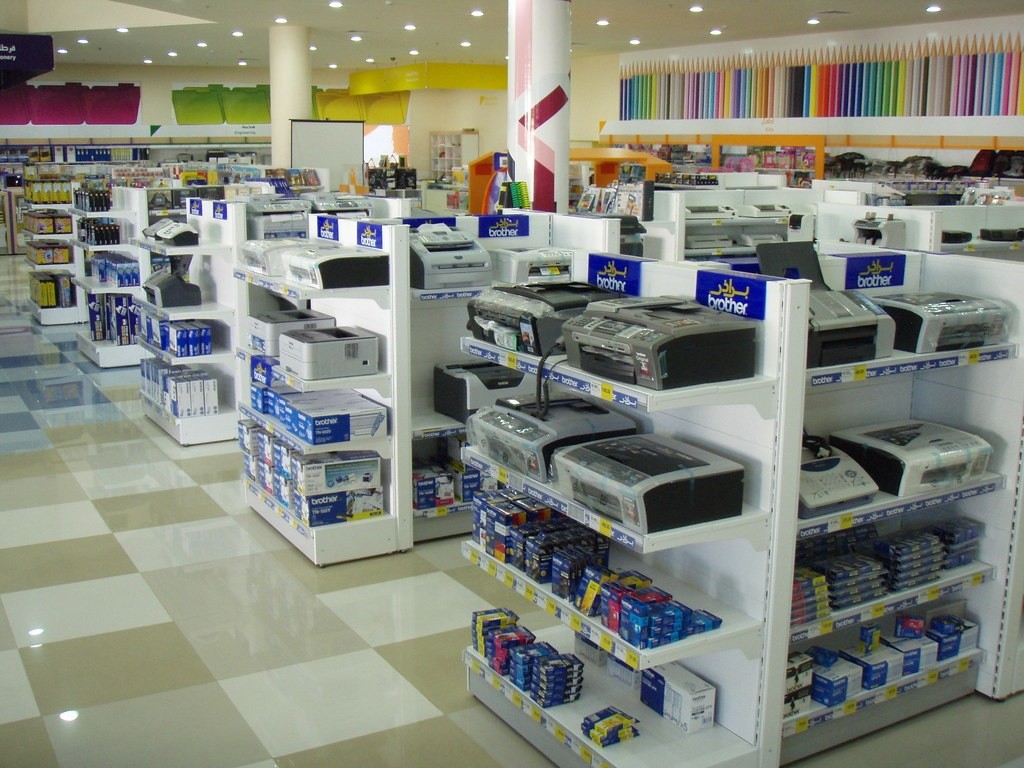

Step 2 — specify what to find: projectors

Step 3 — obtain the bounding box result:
[980,227,1024,241]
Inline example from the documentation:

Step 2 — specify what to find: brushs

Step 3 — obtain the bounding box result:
[509,182,532,210]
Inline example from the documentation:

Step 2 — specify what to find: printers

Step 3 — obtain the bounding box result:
[142,192,1011,537]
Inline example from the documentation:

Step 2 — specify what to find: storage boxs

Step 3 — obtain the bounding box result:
[238,353,386,528]
[412,453,502,519]
[136,306,220,417]
[582,705,640,748]
[473,491,721,649]
[22,214,73,307]
[473,609,584,710]
[82,250,140,346]
[780,515,1001,719]
[640,663,717,732]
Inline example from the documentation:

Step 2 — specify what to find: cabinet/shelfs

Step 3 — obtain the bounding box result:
[20,165,272,326]
[128,180,656,445]
[457,245,1024,768]
[71,184,318,366]
[0,145,271,254]
[237,178,1024,565]
